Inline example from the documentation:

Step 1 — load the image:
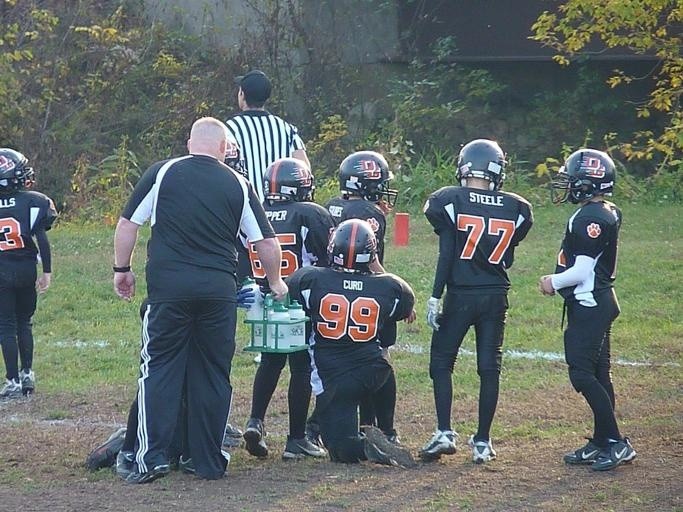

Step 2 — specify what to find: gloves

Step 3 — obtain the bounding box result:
[425,296,442,333]
[237,287,255,309]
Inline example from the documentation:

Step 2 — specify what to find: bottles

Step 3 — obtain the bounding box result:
[250,299,263,347]
[288,300,305,346]
[267,306,274,347]
[271,303,289,349]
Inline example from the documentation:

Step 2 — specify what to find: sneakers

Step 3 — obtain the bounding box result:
[471,438,498,464]
[388,434,400,447]
[1,381,24,399]
[592,440,638,472]
[243,418,272,458]
[86,427,128,471]
[364,423,417,472]
[223,437,241,447]
[418,430,458,461]
[135,463,170,483]
[20,368,37,394]
[116,449,136,481]
[565,441,601,466]
[306,419,325,447]
[282,436,327,460]
[225,423,244,439]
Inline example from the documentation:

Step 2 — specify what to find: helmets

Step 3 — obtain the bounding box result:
[551,166,560,171]
[327,219,377,272]
[0,147,35,199]
[262,158,316,203]
[550,149,616,205]
[337,151,399,208]
[225,133,250,177]
[554,158,559,163]
[455,138,508,192]
[546,158,554,162]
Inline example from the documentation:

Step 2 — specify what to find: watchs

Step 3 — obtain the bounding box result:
[113,265,131,273]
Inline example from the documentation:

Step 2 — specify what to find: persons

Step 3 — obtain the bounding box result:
[419,139,535,467]
[113,117,289,485]
[535,145,639,473]
[235,156,338,461]
[216,70,311,284]
[0,147,53,399]
[114,128,254,482]
[283,217,419,474]
[300,150,417,450]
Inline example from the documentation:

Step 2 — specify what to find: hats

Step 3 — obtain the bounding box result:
[233,71,273,103]
